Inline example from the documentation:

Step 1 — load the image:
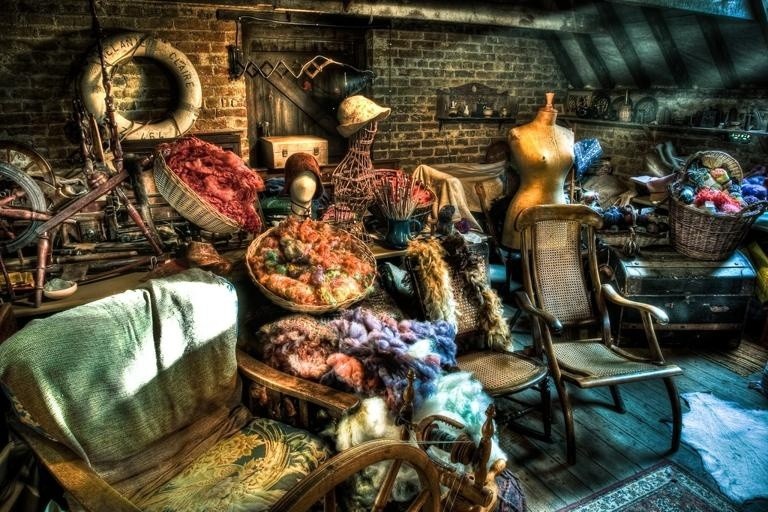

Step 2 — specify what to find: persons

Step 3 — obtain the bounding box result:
[499,92,578,251]
[278,151,325,222]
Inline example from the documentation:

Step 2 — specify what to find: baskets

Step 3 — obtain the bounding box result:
[154,146,243,234]
[247,219,376,316]
[684,151,743,188]
[666,181,768,261]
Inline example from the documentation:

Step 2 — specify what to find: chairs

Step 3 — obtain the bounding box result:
[0,264,361,511]
[404,206,688,467]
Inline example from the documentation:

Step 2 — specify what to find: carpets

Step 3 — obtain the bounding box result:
[554,457,735,512]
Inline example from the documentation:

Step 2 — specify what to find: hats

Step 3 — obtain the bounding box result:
[279,152,324,199]
[336,95,391,137]
